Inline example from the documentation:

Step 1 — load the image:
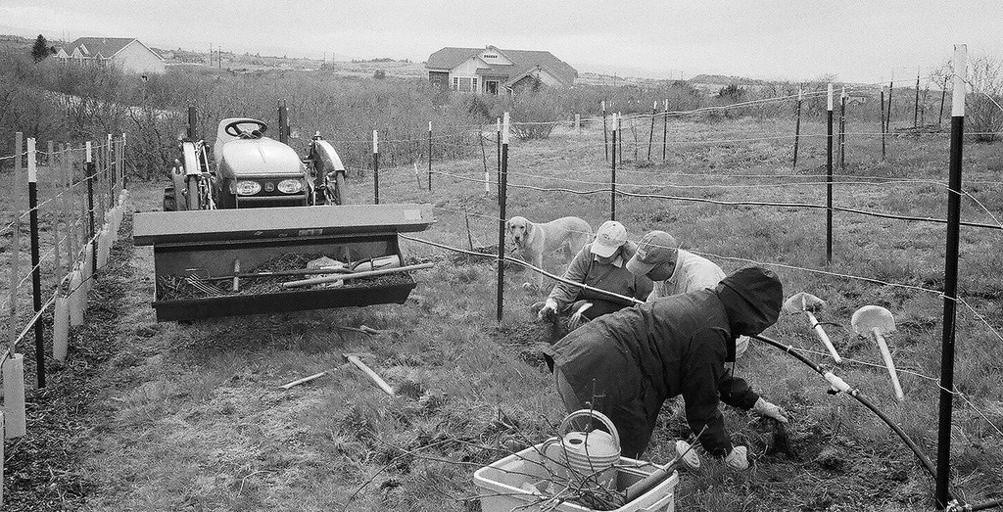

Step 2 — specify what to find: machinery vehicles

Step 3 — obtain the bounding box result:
[133,101,434,322]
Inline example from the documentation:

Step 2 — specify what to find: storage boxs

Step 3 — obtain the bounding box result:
[474,442,679,512]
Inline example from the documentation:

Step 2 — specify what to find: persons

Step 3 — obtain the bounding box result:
[624,231,750,358]
[538,220,653,322]
[545,265,783,471]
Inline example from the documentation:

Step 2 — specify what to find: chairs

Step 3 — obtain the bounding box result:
[214,118,259,167]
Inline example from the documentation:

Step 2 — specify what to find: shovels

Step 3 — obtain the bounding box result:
[782,292,842,364]
[851,305,905,402]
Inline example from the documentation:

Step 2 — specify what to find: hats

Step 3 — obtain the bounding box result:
[590,220,626,257]
[626,230,678,276]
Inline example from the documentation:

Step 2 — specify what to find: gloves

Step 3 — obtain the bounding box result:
[538,298,559,319]
[725,445,750,471]
[753,397,790,426]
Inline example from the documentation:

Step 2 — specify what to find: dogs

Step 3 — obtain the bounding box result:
[505,216,593,291]
